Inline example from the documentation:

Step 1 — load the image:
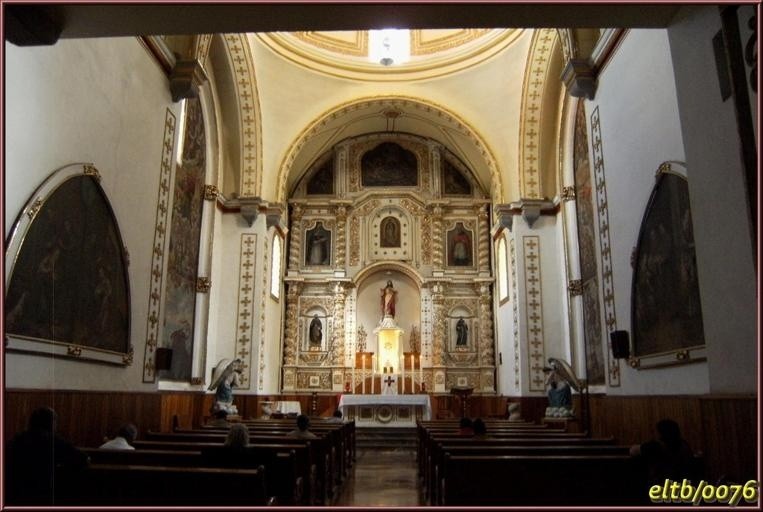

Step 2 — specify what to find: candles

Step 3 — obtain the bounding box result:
[350,355,424,395]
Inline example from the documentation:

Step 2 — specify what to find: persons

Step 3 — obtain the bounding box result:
[381,280,399,321]
[542,366,572,412]
[98,423,138,450]
[630,419,696,507]
[36,219,122,348]
[383,217,398,244]
[456,417,489,438]
[216,368,242,414]
[326,411,344,423]
[452,225,469,261]
[311,232,327,264]
[223,423,250,451]
[285,415,317,439]
[456,316,468,345]
[210,410,231,425]
[635,207,702,331]
[5,406,91,506]
[310,313,322,346]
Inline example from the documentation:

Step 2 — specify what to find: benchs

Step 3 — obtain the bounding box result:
[75,416,355,505]
[417,417,645,504]
